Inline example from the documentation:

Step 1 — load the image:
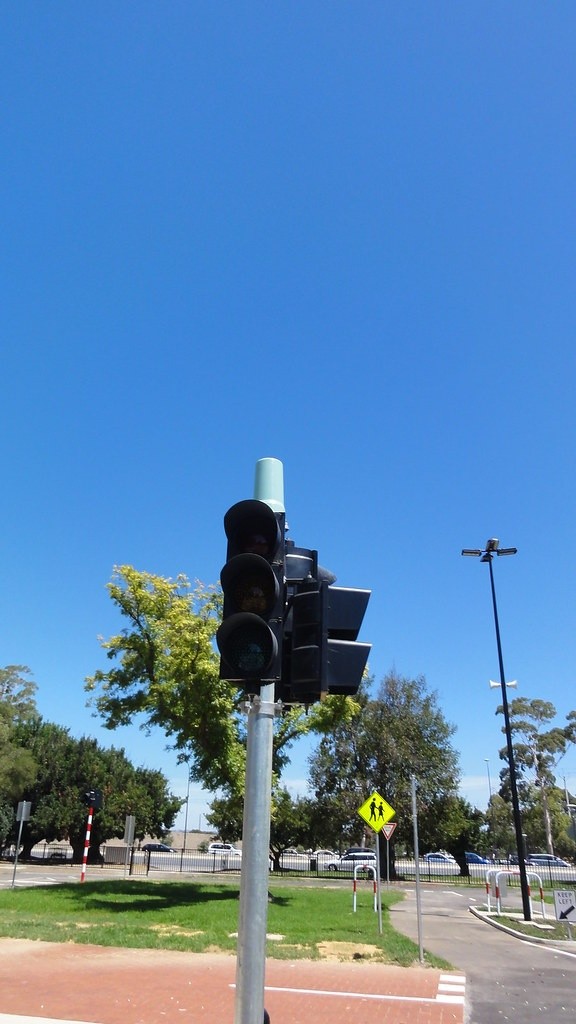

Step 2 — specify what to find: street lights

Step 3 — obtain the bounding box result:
[484,759,493,796]
[460,536,532,922]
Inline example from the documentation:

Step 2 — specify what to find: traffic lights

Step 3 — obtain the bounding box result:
[82,789,101,808]
[215,498,285,685]
[286,577,375,705]
[280,538,337,706]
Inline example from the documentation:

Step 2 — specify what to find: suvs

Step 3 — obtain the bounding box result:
[343,846,377,855]
[526,852,572,867]
[207,842,242,857]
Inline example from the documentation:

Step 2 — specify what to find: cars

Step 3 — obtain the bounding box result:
[309,849,339,860]
[142,843,177,853]
[323,852,377,873]
[499,855,534,866]
[280,848,307,860]
[424,852,456,864]
[455,852,491,864]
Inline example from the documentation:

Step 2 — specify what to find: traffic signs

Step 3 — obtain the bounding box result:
[553,889,576,923]
